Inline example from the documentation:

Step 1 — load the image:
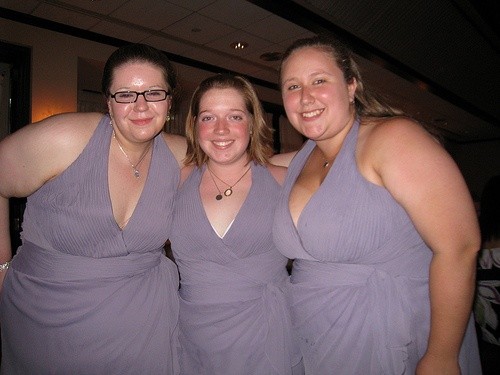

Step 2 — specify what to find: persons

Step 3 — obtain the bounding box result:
[0,44,188,374]
[169,74,305,375]
[268,39,483,375]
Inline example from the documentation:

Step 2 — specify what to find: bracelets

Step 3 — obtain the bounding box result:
[0,261,12,271]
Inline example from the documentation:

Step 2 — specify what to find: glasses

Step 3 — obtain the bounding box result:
[108,89,170,103]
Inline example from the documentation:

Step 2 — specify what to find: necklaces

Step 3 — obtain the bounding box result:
[207,162,251,201]
[319,151,337,167]
[111,126,153,178]
